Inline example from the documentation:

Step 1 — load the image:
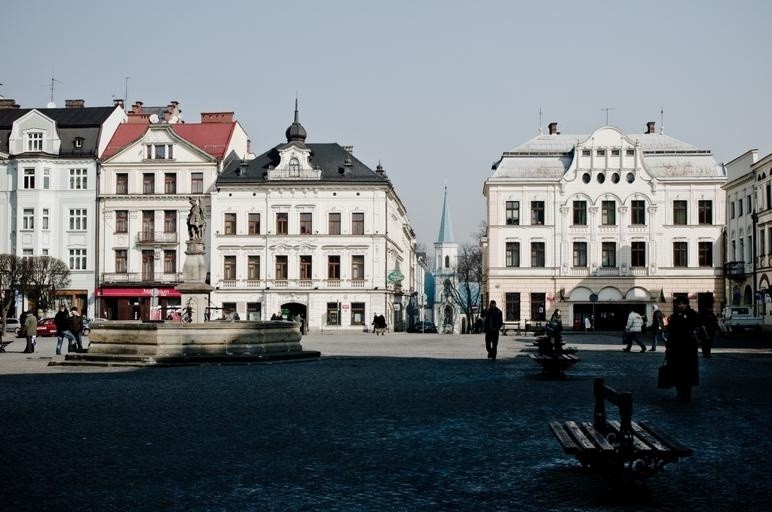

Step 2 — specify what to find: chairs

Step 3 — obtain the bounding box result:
[622,346,656,353]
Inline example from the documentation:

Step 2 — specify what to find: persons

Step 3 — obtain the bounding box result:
[188,197,201,240]
[376,314,387,336]
[648,305,667,351]
[54,305,78,355]
[481,300,502,360]
[372,312,377,333]
[22,311,38,353]
[551,308,562,350]
[664,294,709,403]
[622,306,646,353]
[68,307,84,352]
[295,314,303,322]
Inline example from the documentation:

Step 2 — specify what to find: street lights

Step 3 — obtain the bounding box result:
[658,365,675,387]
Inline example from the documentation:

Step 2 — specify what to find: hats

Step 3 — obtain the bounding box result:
[0,313,105,338]
[414,321,438,333]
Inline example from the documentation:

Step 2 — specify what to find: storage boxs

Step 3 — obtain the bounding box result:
[501,320,695,502]
